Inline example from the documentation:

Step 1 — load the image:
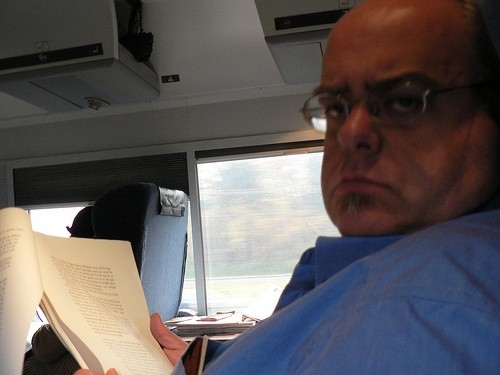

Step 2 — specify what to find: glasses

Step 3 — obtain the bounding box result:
[298,76,487,132]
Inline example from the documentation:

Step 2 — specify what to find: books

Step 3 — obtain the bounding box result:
[162,308,259,336]
[0,203,178,375]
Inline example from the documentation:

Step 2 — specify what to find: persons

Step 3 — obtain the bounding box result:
[65,0,500,375]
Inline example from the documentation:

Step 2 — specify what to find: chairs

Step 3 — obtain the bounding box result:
[91,181,188,321]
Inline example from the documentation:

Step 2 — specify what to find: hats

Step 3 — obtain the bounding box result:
[66,206,93,237]
